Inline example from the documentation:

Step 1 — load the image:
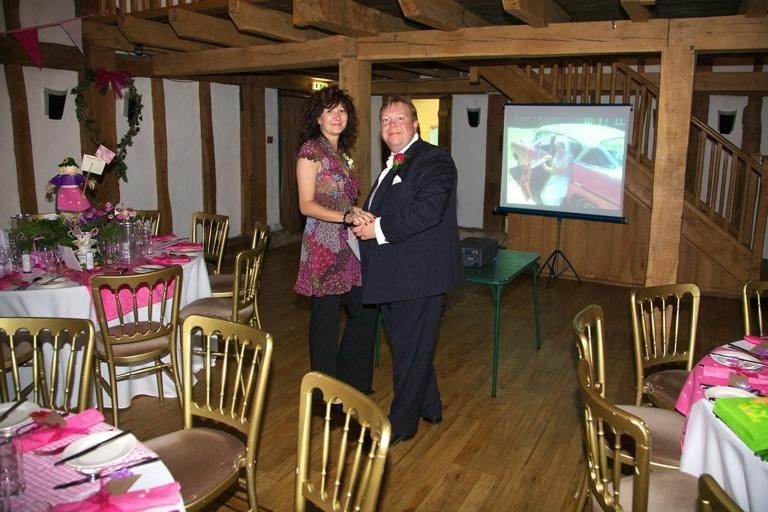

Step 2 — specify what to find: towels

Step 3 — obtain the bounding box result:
[703,364,768,386]
[743,335,762,345]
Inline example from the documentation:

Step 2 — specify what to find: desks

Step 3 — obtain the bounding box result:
[374,248,546,398]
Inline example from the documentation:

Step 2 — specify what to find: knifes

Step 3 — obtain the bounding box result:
[0,398,29,423]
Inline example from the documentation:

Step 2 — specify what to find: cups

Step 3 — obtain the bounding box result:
[1,432,27,496]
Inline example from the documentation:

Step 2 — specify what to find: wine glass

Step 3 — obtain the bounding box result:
[0,249,11,276]
[45,250,64,276]
[11,249,22,272]
[97,241,114,264]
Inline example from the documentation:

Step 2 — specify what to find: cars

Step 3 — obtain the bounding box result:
[512,119,626,209]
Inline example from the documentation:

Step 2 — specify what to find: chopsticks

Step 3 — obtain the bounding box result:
[727,341,762,359]
[53,428,161,491]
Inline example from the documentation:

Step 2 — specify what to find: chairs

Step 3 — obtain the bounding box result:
[741,277,768,341]
[573,356,700,512]
[571,302,687,512]
[695,472,742,512]
[630,281,703,414]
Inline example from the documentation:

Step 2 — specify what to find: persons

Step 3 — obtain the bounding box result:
[46,156,97,224]
[294,85,379,428]
[512,139,537,205]
[541,142,573,205]
[352,97,465,458]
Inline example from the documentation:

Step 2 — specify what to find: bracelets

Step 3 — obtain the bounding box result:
[341,211,349,227]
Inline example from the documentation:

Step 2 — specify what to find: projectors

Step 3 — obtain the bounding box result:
[459,237,498,269]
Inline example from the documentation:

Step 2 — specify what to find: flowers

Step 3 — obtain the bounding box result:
[391,152,409,172]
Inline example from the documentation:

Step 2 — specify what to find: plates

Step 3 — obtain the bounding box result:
[63,431,136,468]
[37,276,70,288]
[168,249,201,257]
[710,351,762,370]
[133,264,167,273]
[0,401,40,430]
[704,386,759,400]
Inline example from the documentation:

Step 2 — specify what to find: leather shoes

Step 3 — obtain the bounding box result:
[362,431,415,449]
[422,416,442,425]
